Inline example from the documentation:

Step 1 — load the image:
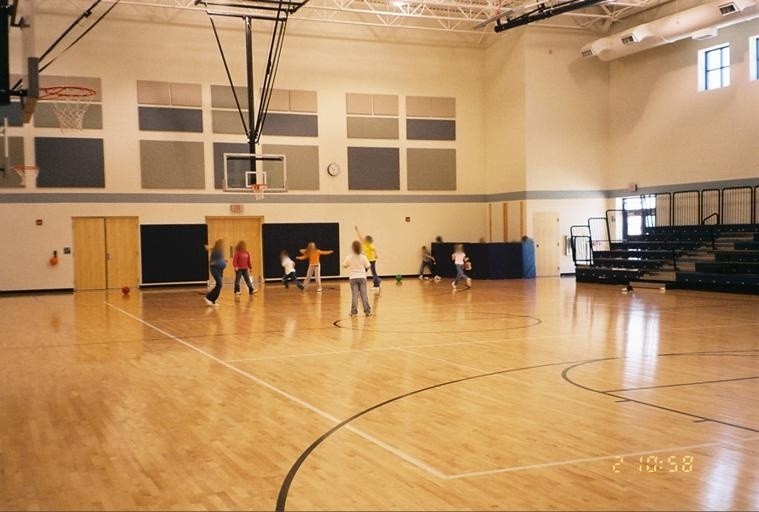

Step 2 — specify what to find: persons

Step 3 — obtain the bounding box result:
[279,248,302,289]
[203,238,230,307]
[296,241,335,292]
[450,243,472,290]
[418,245,436,279]
[232,239,258,296]
[430,235,450,281]
[343,240,373,317]
[354,225,382,289]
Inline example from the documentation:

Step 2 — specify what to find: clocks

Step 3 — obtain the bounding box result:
[328,163,340,176]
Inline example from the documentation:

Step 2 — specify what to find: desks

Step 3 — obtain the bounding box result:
[431,241,536,279]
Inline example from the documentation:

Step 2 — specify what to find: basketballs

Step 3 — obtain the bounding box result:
[122,287,129,294]
[396,274,402,281]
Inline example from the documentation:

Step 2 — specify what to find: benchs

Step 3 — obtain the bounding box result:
[576,224,759,294]
